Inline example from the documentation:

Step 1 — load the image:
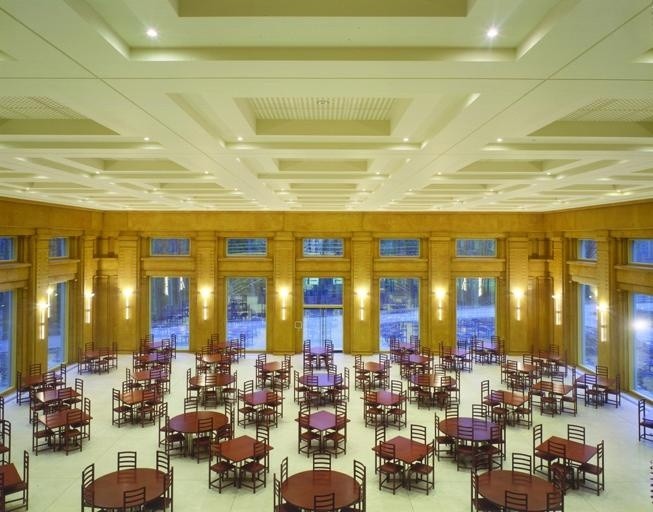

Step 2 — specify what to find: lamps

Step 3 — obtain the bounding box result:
[356,285,369,320]
[201,287,213,322]
[513,288,524,320]
[598,301,610,341]
[278,285,290,322]
[122,287,132,322]
[554,289,565,327]
[433,287,445,321]
[36,298,47,340]
[85,288,94,324]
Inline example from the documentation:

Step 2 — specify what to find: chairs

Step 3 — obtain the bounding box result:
[571,365,621,408]
[1,396,29,512]
[638,397,652,441]
[77,333,607,512]
[1,363,92,457]
